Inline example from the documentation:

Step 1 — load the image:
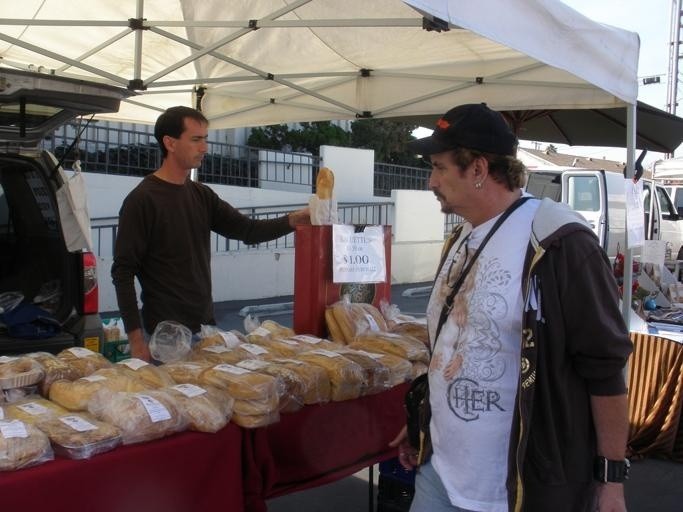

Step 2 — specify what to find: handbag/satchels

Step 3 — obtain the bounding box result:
[613,242,638,278]
[403,372,433,443]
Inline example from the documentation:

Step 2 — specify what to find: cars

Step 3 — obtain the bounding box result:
[0,64,139,360]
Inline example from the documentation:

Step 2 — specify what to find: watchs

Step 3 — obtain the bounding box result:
[593,453,631,485]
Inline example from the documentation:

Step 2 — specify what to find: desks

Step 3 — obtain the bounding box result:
[1,364,415,512]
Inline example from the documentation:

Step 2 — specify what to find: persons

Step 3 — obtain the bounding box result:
[110,102,311,370]
[389,102,639,511]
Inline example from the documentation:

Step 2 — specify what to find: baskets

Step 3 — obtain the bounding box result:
[99,317,131,363]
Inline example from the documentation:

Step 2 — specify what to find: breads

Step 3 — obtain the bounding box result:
[316,166,334,201]
[0,303,430,472]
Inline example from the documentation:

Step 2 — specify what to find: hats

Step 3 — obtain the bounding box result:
[405,102,517,156]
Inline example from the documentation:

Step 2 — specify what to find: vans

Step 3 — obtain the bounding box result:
[524,167,683,270]
